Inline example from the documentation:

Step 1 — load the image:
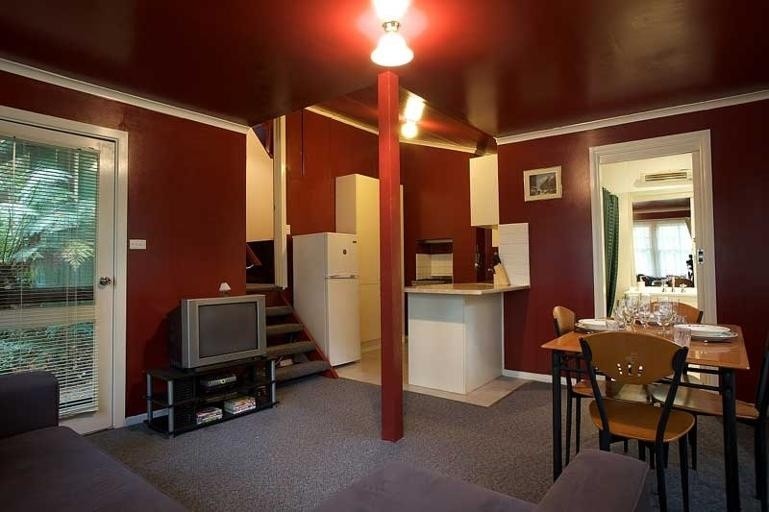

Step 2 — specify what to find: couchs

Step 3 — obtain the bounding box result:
[0,371,651,512]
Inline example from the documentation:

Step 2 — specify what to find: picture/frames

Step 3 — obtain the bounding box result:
[523,165,562,202]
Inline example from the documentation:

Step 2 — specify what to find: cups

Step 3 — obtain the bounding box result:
[673,326,692,348]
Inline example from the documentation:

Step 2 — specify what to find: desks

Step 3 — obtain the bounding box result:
[142,355,280,439]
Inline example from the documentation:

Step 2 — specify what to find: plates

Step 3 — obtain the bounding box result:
[574,318,739,342]
[688,342,739,352]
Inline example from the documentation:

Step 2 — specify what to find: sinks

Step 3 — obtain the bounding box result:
[628,287,695,294]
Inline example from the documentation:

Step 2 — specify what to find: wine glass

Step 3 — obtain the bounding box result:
[614,290,679,346]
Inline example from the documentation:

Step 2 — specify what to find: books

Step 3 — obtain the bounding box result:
[195,406,224,426]
[224,395,257,416]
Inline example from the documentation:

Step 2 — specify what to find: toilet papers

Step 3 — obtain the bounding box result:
[493,263,510,286]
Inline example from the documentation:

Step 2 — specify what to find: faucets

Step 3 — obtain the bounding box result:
[651,276,675,292]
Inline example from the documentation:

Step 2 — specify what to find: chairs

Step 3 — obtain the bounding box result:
[579,331,696,512]
[638,274,651,286]
[552,306,653,467]
[647,350,769,511]
[669,278,694,287]
[638,302,703,471]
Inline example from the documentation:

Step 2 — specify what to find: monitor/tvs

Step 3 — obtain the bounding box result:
[166,294,268,372]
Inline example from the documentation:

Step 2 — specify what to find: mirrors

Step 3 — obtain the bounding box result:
[629,192,697,291]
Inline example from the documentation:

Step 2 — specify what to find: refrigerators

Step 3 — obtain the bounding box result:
[290,232,363,367]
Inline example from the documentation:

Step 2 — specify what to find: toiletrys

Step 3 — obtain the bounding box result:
[637,276,645,292]
